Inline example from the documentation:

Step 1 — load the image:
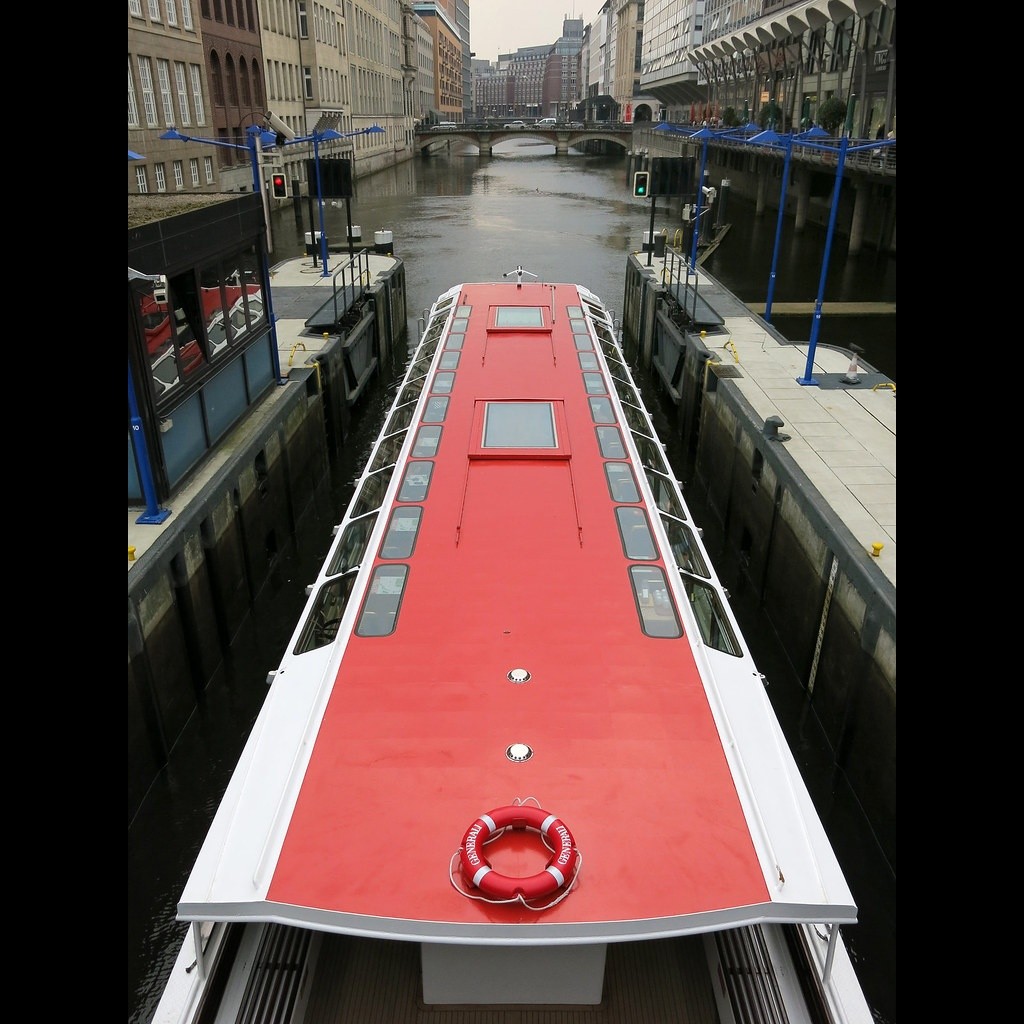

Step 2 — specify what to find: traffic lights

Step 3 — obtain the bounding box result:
[633,172,650,198]
[271,173,289,199]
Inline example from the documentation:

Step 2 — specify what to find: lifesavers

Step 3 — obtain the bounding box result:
[460,799,579,902]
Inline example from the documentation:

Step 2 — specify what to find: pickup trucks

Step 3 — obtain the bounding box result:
[595,122,620,130]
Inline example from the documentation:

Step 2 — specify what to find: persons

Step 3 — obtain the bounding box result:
[690,114,723,127]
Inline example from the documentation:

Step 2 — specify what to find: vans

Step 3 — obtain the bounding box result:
[533,118,557,129]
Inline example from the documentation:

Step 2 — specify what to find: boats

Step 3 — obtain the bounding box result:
[137,268,875,1023]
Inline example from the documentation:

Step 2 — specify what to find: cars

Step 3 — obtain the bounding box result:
[430,121,457,131]
[503,121,527,129]
[560,121,584,129]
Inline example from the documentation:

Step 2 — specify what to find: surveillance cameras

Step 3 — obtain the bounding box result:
[153,288,168,304]
[702,186,711,195]
[263,111,295,141]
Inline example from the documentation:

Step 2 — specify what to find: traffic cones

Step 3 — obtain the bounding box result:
[840,352,862,386]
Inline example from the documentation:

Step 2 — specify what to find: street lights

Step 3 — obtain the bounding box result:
[156,127,342,320]
[691,127,829,320]
[653,123,763,276]
[747,130,896,387]
[247,123,386,277]
